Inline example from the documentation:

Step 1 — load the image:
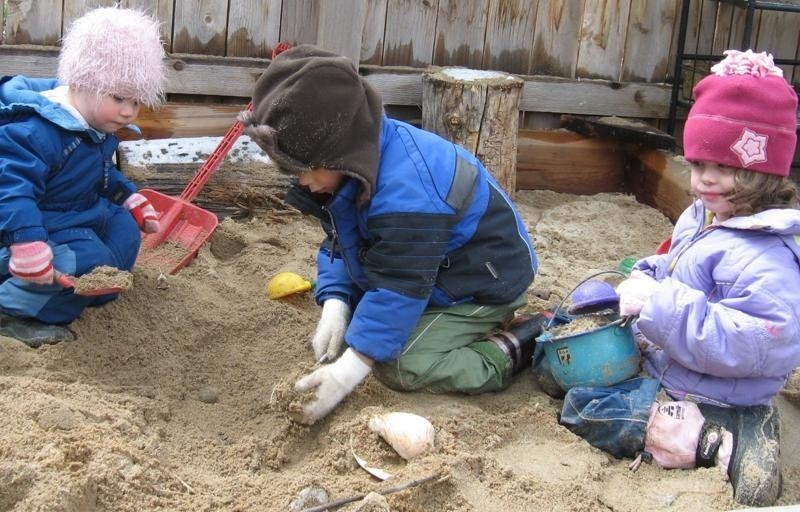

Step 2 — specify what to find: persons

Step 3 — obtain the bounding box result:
[530,50,799,506]
[238,45,581,427]
[0,4,168,349]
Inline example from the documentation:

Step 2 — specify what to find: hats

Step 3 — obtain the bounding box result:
[683,50,799,179]
[60,6,164,109]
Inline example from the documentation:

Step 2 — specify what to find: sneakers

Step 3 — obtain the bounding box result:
[1,318,76,349]
[701,406,783,506]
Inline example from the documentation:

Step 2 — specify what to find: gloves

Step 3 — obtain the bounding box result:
[294,349,372,424]
[122,192,160,234]
[7,240,55,286]
[615,270,660,317]
[311,298,353,363]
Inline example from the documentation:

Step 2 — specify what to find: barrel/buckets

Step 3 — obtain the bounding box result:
[535,267,643,394]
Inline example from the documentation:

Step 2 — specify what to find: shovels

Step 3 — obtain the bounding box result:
[134,41,297,275]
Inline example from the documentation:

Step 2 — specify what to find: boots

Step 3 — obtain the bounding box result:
[489,305,576,378]
